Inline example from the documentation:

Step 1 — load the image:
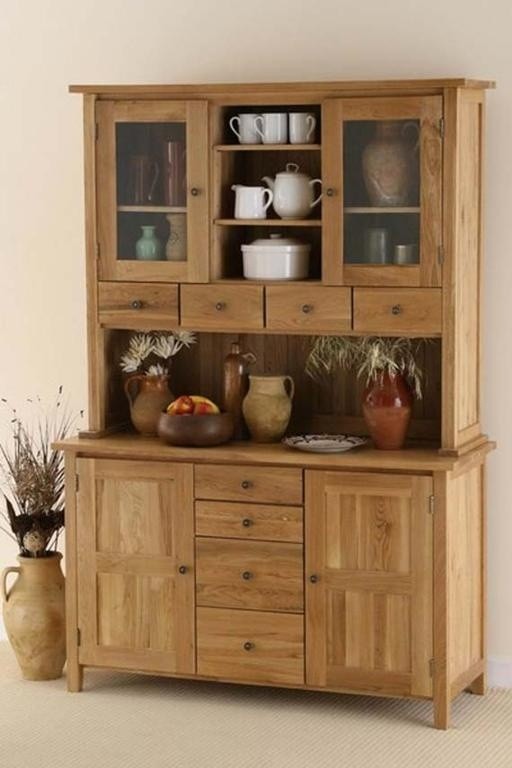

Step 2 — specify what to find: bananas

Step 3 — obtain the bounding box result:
[167,396,221,413]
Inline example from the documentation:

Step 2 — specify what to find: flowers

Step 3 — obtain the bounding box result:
[120,330,198,376]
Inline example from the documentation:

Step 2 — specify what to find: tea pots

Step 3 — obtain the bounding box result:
[261,163,324,220]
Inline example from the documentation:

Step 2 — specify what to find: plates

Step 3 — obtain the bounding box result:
[281,432,367,454]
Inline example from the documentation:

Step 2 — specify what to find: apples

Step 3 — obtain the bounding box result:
[174,396,193,412]
[192,402,213,413]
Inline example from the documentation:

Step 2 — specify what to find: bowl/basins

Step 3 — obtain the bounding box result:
[157,409,235,447]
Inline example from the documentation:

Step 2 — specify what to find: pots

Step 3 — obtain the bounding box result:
[240,233,312,280]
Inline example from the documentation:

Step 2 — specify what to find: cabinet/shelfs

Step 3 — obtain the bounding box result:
[50,443,196,676]
[303,443,494,701]
[195,457,301,687]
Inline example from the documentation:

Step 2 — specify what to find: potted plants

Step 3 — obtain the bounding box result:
[0,380,86,681]
[306,337,438,450]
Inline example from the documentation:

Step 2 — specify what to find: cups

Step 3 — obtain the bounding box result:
[253,113,288,144]
[289,112,316,144]
[233,186,273,220]
[161,141,185,207]
[124,154,159,205]
[229,113,261,144]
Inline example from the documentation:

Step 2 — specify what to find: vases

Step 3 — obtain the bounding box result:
[242,371,296,444]
